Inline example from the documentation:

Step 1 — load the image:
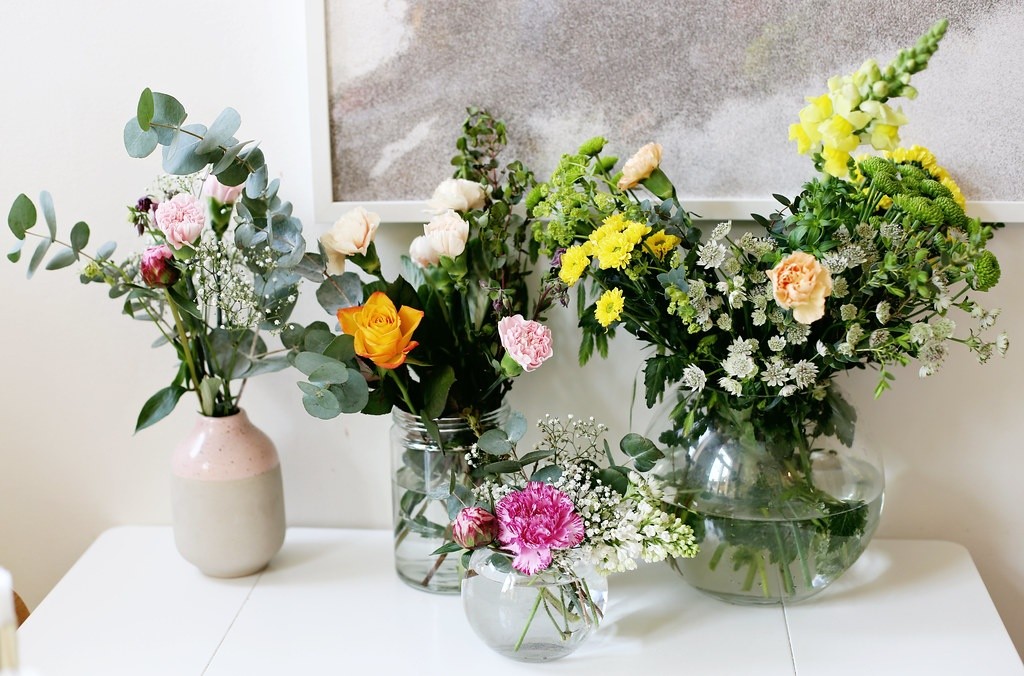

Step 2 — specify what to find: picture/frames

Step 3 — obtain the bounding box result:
[304,0,1024,224]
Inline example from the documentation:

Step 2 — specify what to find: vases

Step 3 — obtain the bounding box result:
[180,405,288,578]
[461,543,606,665]
[642,379,887,604]
[388,404,513,594]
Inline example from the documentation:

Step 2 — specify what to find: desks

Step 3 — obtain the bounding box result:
[16,526,1024,676]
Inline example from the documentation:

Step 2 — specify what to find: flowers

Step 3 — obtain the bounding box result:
[8,19,1010,652]
